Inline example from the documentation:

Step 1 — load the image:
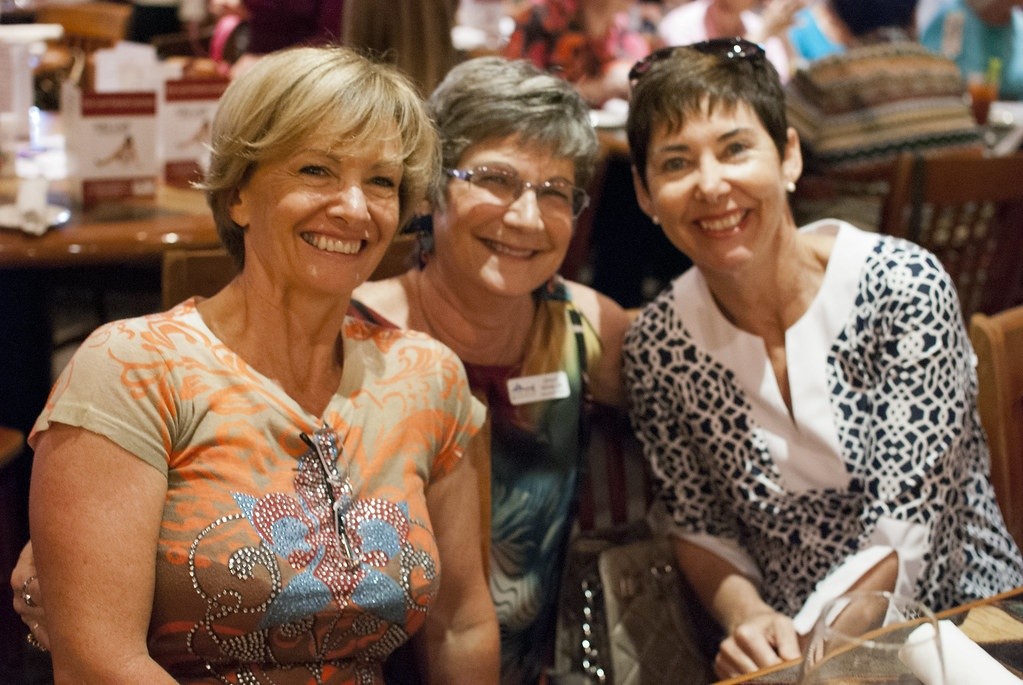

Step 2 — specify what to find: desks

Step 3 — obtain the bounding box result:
[0,183,221,269]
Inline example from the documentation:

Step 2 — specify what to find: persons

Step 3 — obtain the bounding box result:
[622,37,1022,685]
[447,0,1022,110]
[25,45,501,685]
[12,56,633,685]
[208,0,324,79]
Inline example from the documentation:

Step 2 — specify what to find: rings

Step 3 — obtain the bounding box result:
[20,575,38,607]
[26,623,46,652]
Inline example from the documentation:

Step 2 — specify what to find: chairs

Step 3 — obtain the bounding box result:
[160,145,1023,555]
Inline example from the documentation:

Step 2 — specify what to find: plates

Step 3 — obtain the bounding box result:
[0,201,71,228]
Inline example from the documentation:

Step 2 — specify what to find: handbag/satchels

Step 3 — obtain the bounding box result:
[550,525,715,685]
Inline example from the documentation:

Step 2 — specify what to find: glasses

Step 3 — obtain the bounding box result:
[301,420,367,568]
[441,163,590,221]
[628,36,766,96]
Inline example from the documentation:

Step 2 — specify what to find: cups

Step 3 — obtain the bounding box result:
[14,145,49,222]
[967,70,999,124]
[795,590,948,685]
